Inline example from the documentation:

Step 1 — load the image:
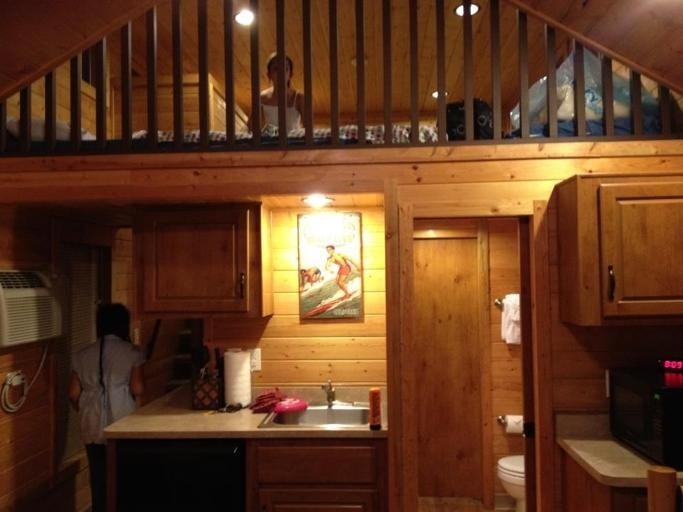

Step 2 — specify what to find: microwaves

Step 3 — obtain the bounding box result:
[608,368,682,465]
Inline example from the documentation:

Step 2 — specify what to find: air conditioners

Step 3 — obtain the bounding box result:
[0,269,62,349]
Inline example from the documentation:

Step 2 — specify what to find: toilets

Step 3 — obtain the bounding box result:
[497,455,527,512]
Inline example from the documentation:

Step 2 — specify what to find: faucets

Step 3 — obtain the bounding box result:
[321,379,336,405]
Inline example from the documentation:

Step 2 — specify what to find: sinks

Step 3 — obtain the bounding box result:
[257,402,371,428]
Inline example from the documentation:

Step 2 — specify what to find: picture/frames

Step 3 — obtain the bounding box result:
[296,211,362,320]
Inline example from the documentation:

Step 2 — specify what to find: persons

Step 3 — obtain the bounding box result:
[322,242,360,300]
[301,266,323,291]
[246,51,308,132]
[67,301,147,511]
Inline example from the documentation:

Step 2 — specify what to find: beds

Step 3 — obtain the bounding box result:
[3,117,437,151]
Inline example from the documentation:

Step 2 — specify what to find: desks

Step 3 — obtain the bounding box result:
[556,436,682,511]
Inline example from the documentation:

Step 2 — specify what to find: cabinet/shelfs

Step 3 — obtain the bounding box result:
[140,203,276,318]
[247,437,384,511]
[554,172,682,327]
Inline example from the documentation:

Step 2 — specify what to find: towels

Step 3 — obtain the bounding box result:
[500,292,523,347]
